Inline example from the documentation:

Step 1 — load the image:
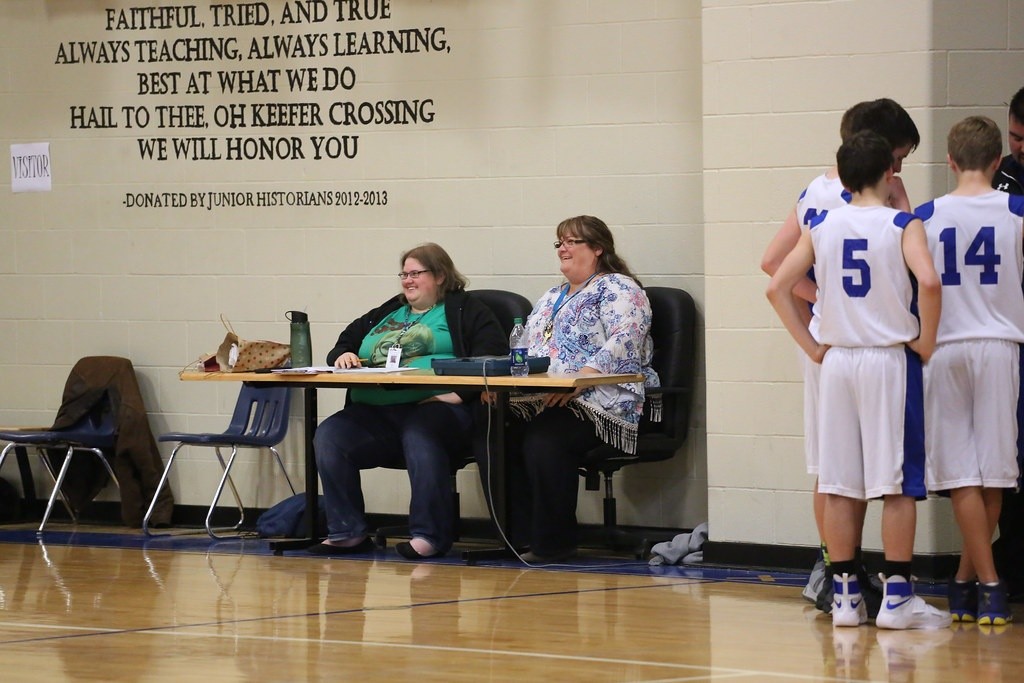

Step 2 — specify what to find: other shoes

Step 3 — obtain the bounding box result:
[396,538,452,561]
[520,545,576,564]
[815,567,883,618]
[308,534,371,555]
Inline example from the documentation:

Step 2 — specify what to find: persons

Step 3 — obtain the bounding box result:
[760,81,1024,631]
[307,242,510,561]
[471,214,663,564]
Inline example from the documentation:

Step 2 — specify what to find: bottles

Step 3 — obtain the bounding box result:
[285,311,313,368]
[509,318,530,376]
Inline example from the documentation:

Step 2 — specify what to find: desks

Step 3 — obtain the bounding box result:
[181,370,644,565]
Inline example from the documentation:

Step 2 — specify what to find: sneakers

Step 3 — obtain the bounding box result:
[875,572,952,629]
[976,581,1013,626]
[833,573,868,626]
[802,561,825,601]
[948,578,975,622]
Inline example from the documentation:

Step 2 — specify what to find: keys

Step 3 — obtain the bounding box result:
[542,331,551,346]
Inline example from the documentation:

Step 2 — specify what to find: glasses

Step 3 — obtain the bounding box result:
[554,237,593,247]
[399,268,436,277]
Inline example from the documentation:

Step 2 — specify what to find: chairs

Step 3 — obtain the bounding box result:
[373,291,533,547]
[574,288,696,558]
[142,385,295,540]
[0,357,132,538]
